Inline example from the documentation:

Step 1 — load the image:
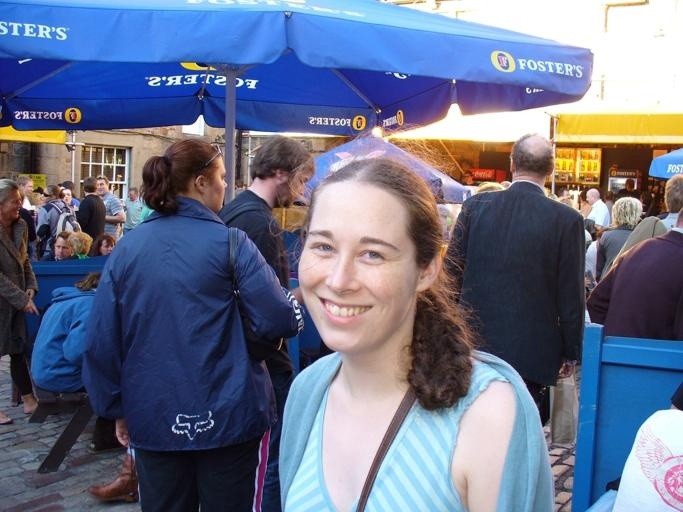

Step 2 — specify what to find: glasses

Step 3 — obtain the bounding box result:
[40,191,50,196]
[194,143,223,186]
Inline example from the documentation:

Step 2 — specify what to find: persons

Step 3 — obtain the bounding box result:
[222,136,298,505]
[111,185,145,236]
[69,233,93,260]
[92,235,113,256]
[596,197,642,291]
[95,176,124,238]
[586,372,683,512]
[616,182,636,203]
[441,135,586,425]
[583,186,608,236]
[458,171,476,186]
[60,191,77,215]
[278,158,558,510]
[73,177,105,236]
[57,180,78,200]
[589,206,681,343]
[0,178,41,425]
[33,274,134,452]
[16,189,35,256]
[611,178,683,267]
[12,177,36,215]
[35,185,74,259]
[82,139,309,508]
[34,233,71,260]
[639,192,660,214]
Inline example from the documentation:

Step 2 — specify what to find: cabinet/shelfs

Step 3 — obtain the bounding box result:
[546,147,601,185]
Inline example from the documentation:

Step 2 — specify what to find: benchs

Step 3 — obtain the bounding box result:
[21,361,91,473]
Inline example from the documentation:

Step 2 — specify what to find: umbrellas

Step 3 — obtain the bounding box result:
[0,0,593,219]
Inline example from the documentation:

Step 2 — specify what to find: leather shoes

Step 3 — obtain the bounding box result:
[1,413,10,422]
[22,394,44,412]
[88,449,139,503]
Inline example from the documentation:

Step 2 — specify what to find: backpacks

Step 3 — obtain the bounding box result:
[47,201,81,238]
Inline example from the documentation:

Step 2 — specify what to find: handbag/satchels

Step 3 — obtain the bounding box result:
[229,225,282,363]
[548,359,577,444]
